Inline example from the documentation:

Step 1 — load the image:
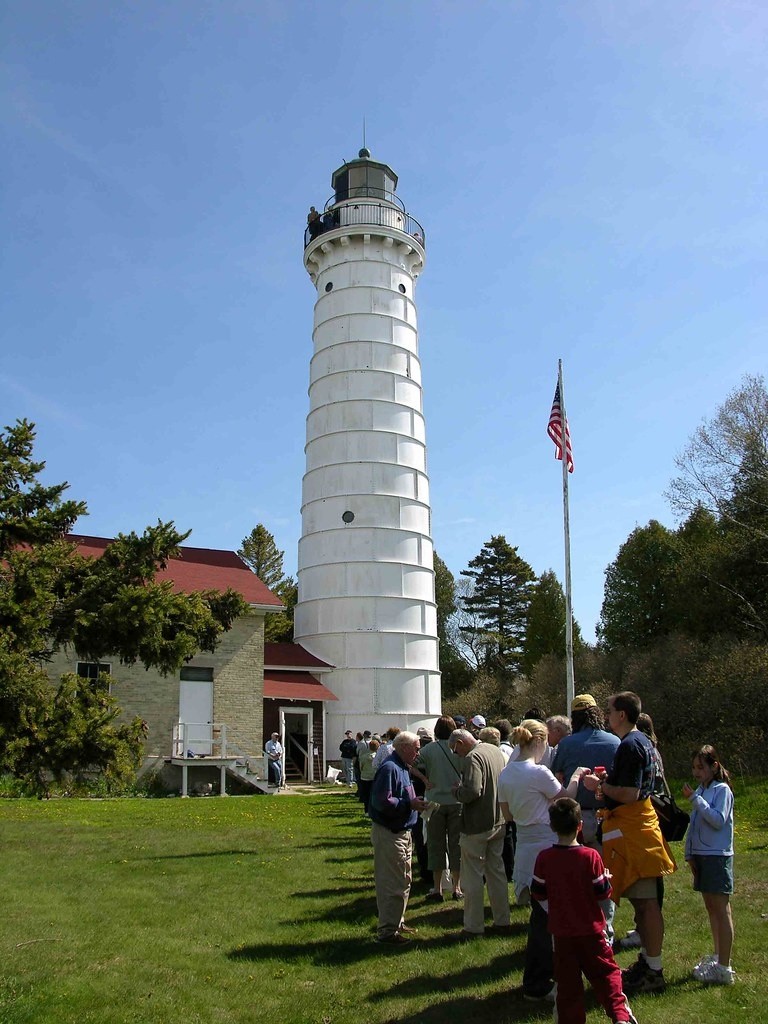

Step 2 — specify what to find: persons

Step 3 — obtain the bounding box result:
[550,694,621,948]
[339,730,356,788]
[681,744,735,985]
[354,707,574,887]
[368,730,430,945]
[531,797,639,1024]
[582,691,678,991]
[408,715,465,902]
[615,713,665,945]
[498,720,591,1003]
[265,732,283,787]
[446,729,511,936]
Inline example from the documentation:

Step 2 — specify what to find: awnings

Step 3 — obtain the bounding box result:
[263,669,340,702]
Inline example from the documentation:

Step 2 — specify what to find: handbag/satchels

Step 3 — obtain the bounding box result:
[651,795,689,842]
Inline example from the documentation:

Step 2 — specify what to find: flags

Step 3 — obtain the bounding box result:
[547,377,574,473]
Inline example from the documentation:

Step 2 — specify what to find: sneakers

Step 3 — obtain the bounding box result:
[694,954,719,977]
[621,954,666,992]
[696,965,734,984]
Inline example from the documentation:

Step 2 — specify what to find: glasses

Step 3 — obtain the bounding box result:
[346,733,351,734]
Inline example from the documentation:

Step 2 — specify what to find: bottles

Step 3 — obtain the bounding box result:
[594,766,607,801]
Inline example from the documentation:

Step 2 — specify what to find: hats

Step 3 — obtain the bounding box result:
[417,727,429,737]
[572,694,597,711]
[469,715,487,728]
[271,732,279,738]
[345,730,352,735]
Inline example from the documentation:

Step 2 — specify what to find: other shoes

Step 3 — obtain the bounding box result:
[349,784,352,788]
[621,936,643,946]
[626,928,640,937]
[524,980,557,1002]
[398,924,414,933]
[350,793,357,797]
[452,929,484,940]
[492,924,511,933]
[426,892,444,900]
[365,813,369,817]
[381,933,410,944]
[453,892,464,900]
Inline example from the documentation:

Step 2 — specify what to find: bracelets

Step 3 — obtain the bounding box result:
[596,781,604,793]
